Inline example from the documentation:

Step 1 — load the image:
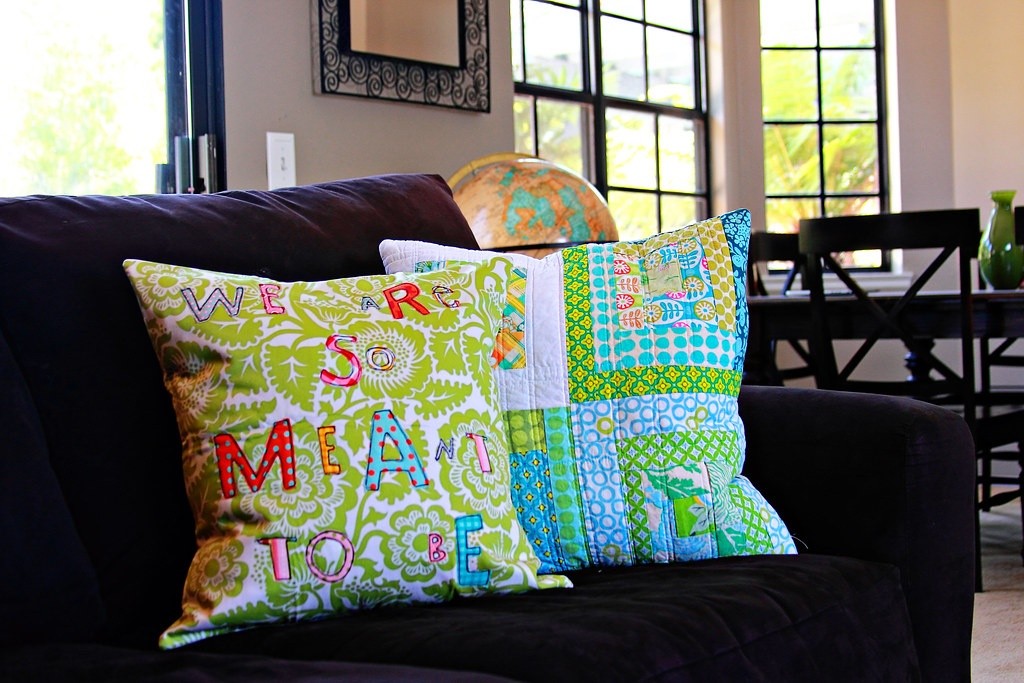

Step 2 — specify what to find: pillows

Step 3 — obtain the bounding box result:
[120,256,578,652]
[375,208,799,573]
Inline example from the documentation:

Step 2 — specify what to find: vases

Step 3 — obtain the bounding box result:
[979,190,1024,290]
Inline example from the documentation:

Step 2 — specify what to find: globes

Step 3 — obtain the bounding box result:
[447,152,619,249]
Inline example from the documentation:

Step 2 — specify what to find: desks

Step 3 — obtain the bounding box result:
[745,290,1023,567]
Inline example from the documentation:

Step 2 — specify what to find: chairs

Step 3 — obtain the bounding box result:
[794,207,1024,594]
[978,229,1024,536]
[739,232,836,388]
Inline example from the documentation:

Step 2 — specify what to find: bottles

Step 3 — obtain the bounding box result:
[979,190,1024,291]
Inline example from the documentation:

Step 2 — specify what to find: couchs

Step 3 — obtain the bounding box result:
[0,169,980,680]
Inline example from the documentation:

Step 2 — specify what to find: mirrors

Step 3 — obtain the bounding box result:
[315,0,491,113]
